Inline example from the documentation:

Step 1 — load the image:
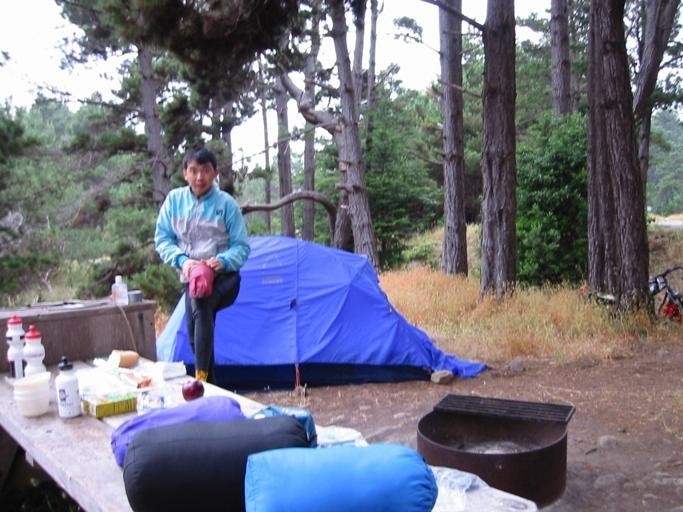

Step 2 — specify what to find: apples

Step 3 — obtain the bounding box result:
[182,379,204,402]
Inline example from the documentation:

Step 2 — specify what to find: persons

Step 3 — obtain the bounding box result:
[154,148,250,384]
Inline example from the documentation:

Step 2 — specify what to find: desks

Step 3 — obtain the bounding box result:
[0,351,540,512]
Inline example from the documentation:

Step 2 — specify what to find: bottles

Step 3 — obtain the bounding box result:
[23,322,49,376]
[6,314,26,380]
[111,275,127,308]
[55,355,84,419]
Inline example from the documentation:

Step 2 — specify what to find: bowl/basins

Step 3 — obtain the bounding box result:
[14,372,52,418]
[128,291,142,303]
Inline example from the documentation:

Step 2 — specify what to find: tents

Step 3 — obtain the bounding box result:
[157,236,495,394]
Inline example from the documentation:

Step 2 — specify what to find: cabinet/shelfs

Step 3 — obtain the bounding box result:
[1,292,160,362]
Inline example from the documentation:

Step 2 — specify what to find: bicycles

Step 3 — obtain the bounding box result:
[594,264,683,325]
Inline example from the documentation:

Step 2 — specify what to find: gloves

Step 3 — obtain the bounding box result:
[182,260,202,279]
[205,257,227,272]
